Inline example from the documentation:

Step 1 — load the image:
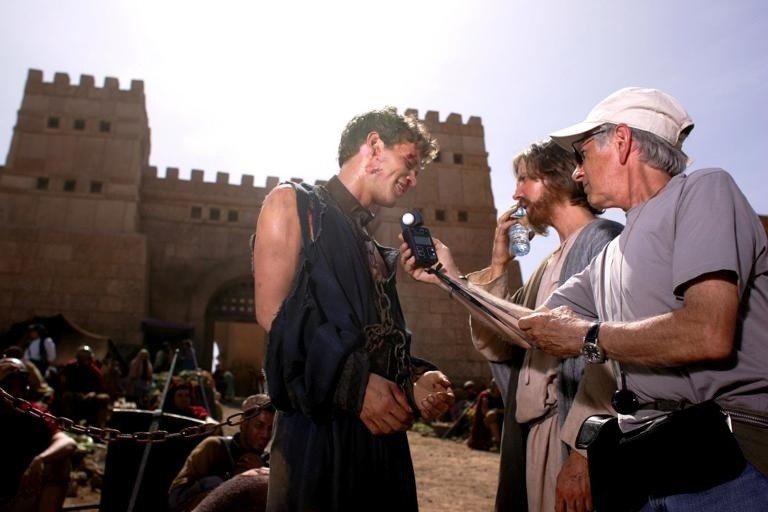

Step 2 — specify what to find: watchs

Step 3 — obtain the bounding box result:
[580,318,606,367]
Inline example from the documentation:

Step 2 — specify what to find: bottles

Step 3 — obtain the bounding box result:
[507,206,532,257]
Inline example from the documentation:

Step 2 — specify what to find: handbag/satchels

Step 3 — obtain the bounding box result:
[574,400,746,511]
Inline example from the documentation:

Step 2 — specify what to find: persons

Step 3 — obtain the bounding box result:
[166,392,276,512]
[0,324,194,512]
[248,103,456,512]
[212,364,227,405]
[223,368,235,402]
[449,379,475,419]
[398,84,767,512]
[471,378,502,450]
[461,137,626,512]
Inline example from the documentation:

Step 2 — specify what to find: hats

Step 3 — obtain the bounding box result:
[548,86,694,154]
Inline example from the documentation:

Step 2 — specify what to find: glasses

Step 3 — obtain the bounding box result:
[571,128,609,164]
